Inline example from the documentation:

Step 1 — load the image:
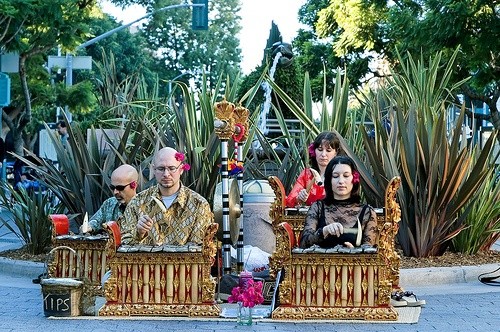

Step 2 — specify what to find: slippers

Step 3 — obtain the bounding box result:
[392,291,426,306]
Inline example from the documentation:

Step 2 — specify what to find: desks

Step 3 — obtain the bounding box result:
[102,220,219,318]
[269,174,406,323]
[50,236,109,289]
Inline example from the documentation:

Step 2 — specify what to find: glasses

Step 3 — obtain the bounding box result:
[153,162,182,173]
[110,184,130,191]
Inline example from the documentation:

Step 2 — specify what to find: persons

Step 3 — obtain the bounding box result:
[79,164,140,237]
[119,147,212,246]
[286,132,341,207]
[57,120,72,174]
[300,157,377,248]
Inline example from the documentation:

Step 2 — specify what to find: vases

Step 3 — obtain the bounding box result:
[237,302,252,326]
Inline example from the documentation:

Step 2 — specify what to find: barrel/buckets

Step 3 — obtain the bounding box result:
[41,278,82,317]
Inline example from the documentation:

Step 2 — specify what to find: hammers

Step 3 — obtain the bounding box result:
[343,216,362,246]
[299,168,322,203]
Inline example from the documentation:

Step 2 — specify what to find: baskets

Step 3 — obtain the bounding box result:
[40,245,84,317]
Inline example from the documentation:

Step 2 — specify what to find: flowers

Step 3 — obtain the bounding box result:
[351,171,360,184]
[130,182,136,189]
[175,153,190,171]
[228,279,265,308]
[308,143,316,158]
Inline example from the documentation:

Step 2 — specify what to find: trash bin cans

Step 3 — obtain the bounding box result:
[243,179,276,255]
